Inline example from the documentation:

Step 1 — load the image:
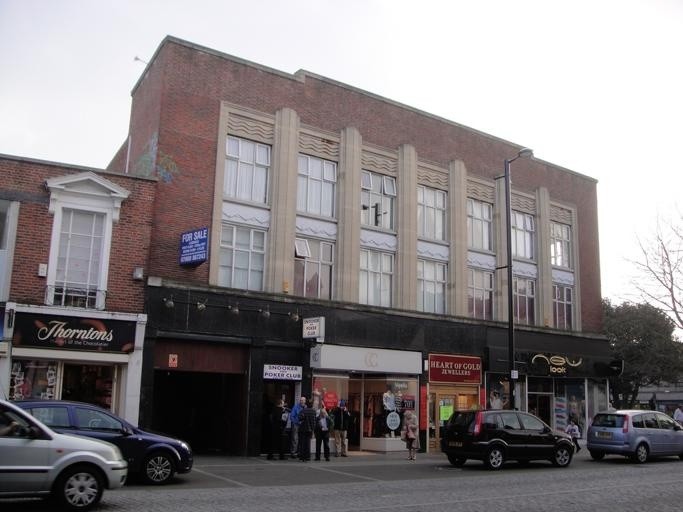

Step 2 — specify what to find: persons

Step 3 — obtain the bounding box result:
[565,418,581,453]
[264,398,288,460]
[308,386,329,411]
[567,395,584,427]
[0,420,19,435]
[663,405,682,429]
[393,390,403,413]
[313,407,333,462]
[297,399,316,461]
[489,390,509,409]
[401,410,419,460]
[382,390,396,438]
[289,396,307,459]
[330,399,352,457]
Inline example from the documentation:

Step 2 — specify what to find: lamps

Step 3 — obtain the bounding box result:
[162,290,304,324]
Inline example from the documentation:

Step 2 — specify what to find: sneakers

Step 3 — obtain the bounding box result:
[334,454,348,457]
[407,456,416,460]
[314,457,330,461]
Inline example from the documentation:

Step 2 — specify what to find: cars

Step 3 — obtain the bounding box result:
[4,397,196,484]
[0,399,128,509]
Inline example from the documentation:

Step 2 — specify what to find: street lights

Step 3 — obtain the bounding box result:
[505,146,533,409]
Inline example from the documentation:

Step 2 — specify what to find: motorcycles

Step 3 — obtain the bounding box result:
[565,430,578,455]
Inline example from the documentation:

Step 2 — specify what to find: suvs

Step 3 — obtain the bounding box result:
[440,409,579,467]
[588,408,682,461]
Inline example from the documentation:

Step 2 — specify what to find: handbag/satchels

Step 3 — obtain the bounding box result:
[407,431,416,440]
[401,431,409,441]
